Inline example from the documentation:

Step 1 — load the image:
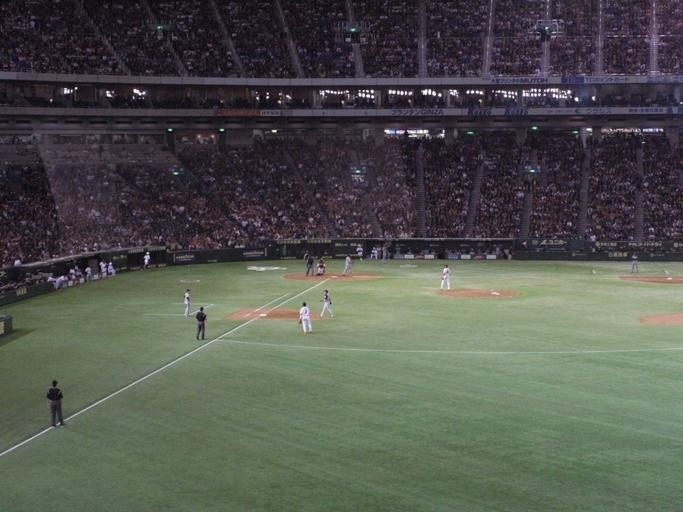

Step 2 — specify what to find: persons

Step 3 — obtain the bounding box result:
[318,290,336,319]
[0,1,683,299]
[297,301,313,333]
[45,379,63,428]
[181,286,192,317]
[195,306,206,341]
